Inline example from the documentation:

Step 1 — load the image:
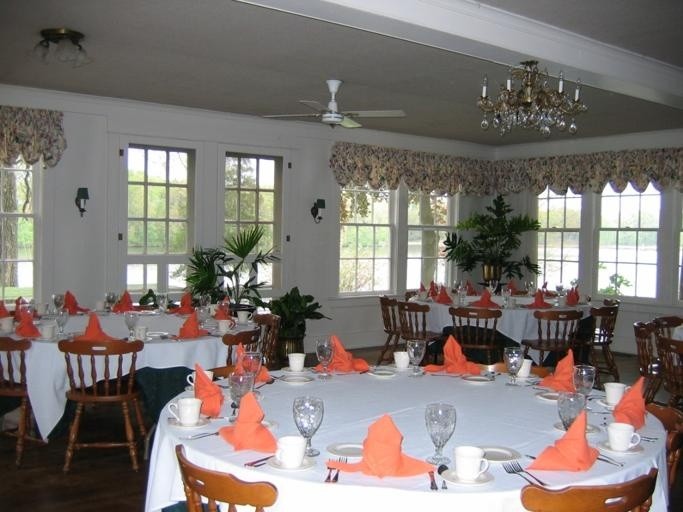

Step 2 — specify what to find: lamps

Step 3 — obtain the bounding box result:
[442,194,541,282]
[311,199,325,224]
[31,28,91,68]
[478,60,588,137]
[75,188,89,217]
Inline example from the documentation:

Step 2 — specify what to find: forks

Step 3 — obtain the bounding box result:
[502,460,549,488]
[323,456,349,484]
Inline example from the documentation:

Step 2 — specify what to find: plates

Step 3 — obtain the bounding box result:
[279,365,311,374]
[552,419,603,436]
[597,397,618,409]
[439,468,496,487]
[266,456,319,473]
[462,373,494,384]
[595,438,647,455]
[326,441,365,459]
[139,311,156,316]
[535,391,562,402]
[210,329,233,336]
[278,374,313,385]
[166,418,211,429]
[571,364,596,412]
[184,384,199,393]
[476,445,523,463]
[367,368,398,378]
[509,373,538,380]
[145,330,169,337]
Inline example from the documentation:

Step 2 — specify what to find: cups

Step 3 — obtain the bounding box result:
[287,352,306,372]
[606,422,642,451]
[274,435,307,468]
[392,351,412,368]
[517,357,532,377]
[0,316,14,333]
[185,370,213,389]
[136,325,148,341]
[34,302,55,338]
[166,396,202,426]
[210,303,253,330]
[452,445,491,483]
[603,381,633,404]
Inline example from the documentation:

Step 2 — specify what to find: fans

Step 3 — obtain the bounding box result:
[262,80,406,128]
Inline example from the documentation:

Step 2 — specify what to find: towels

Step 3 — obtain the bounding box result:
[419,279,556,300]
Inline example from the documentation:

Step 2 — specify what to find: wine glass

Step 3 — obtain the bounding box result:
[502,347,524,386]
[407,339,427,375]
[423,402,457,466]
[241,351,263,375]
[228,371,256,423]
[52,291,139,341]
[312,337,336,380]
[292,395,329,459]
[558,390,587,432]
[451,277,567,307]
[155,293,167,316]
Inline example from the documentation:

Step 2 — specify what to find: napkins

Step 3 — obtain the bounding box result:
[112,290,141,312]
[59,290,91,315]
[232,342,273,383]
[324,412,438,479]
[212,303,232,320]
[313,334,369,374]
[217,390,279,454]
[2,302,10,318]
[611,376,650,431]
[167,292,196,315]
[72,311,114,341]
[192,362,226,418]
[13,296,44,338]
[524,408,600,474]
[222,296,231,305]
[172,310,210,340]
[424,334,482,376]
[535,348,578,393]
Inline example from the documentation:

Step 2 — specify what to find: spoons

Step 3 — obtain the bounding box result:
[437,462,449,489]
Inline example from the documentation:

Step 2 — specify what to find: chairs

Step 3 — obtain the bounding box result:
[630,314,683,407]
[589,298,621,390]
[54,337,150,474]
[208,312,281,379]
[174,443,278,511]
[520,309,584,367]
[375,294,444,366]
[1,336,46,471]
[518,467,658,512]
[645,402,683,497]
[447,306,503,364]
